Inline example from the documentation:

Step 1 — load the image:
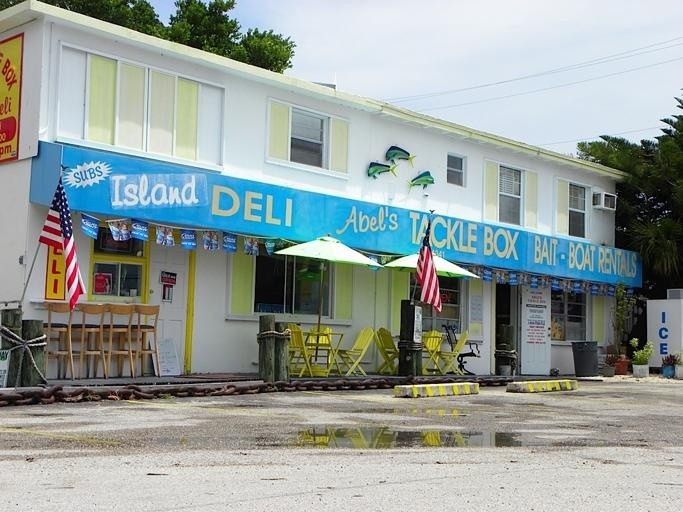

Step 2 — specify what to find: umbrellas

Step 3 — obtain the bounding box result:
[383,244,479,333]
[275,233,384,367]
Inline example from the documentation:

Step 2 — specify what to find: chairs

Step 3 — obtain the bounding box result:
[42,301,161,380]
[283,324,482,379]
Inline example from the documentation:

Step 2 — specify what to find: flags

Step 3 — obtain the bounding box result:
[78,210,260,257]
[37,178,87,312]
[414,218,444,314]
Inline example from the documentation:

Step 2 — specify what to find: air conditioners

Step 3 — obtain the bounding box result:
[594,191,617,213]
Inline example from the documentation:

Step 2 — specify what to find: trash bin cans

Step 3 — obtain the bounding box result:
[571,342,599,376]
[495,349,517,376]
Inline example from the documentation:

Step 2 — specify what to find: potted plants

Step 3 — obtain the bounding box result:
[604,338,683,380]
[611,279,635,374]
